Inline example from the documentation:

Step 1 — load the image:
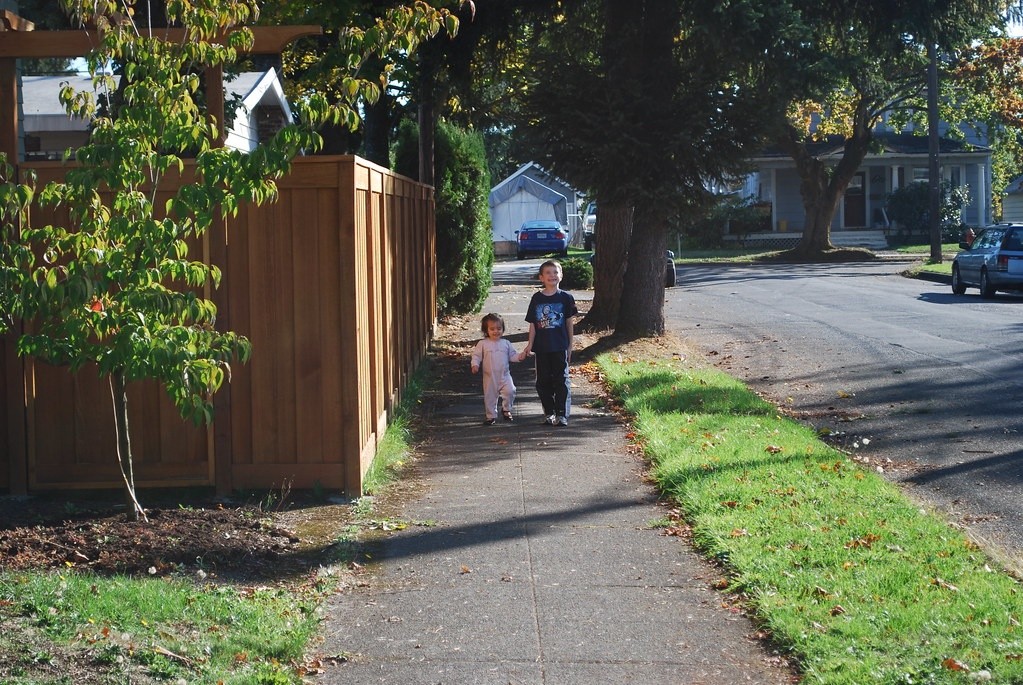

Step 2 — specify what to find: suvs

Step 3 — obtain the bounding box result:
[582,203,597,252]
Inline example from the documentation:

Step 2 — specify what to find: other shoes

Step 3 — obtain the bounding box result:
[484,418,495,425]
[501,408,513,422]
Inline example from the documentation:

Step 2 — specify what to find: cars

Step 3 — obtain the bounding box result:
[514,220,569,260]
[666,248,677,286]
[951,222,1023,299]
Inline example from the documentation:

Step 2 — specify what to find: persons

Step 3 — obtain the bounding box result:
[470,313,527,426]
[524,260,578,428]
[965,227,976,249]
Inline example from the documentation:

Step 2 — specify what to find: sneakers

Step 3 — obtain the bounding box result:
[556,415,568,426]
[544,414,556,424]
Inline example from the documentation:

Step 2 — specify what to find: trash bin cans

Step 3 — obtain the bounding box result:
[779,221,788,231]
